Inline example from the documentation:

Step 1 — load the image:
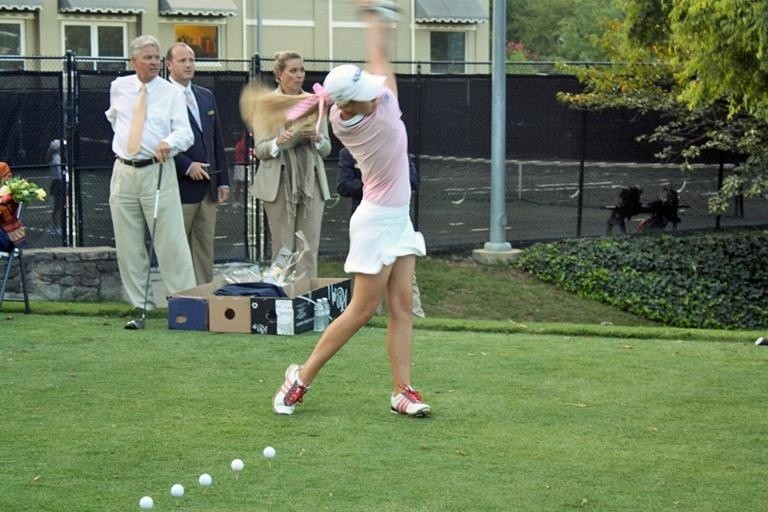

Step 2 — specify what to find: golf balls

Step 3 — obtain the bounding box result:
[170,483,185,498]
[231,459,244,471]
[139,496,153,510]
[263,446,275,458]
[198,474,213,487]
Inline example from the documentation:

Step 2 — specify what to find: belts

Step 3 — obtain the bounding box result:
[116,156,160,168]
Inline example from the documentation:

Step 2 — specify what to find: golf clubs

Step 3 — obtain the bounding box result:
[123,162,166,331]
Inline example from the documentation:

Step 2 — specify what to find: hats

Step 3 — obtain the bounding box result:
[323,63,389,109]
[49,138,68,151]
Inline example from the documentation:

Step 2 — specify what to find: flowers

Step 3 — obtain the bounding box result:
[1,176,47,204]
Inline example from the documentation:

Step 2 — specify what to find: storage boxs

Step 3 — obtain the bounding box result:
[250,277,353,337]
[168,278,234,331]
[209,279,305,333]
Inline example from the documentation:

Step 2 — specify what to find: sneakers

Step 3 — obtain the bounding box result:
[390,382,433,419]
[271,363,313,416]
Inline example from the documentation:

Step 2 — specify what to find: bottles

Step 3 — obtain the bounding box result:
[322,298,330,325]
[314,299,325,332]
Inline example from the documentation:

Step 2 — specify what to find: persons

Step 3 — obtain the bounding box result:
[239,0,434,418]
[103,33,196,318]
[0,161,30,254]
[250,49,332,280]
[162,42,231,285]
[234,129,250,203]
[337,143,427,318]
[607,184,679,236]
[45,138,64,236]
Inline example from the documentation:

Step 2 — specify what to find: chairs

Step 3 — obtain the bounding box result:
[0,200,33,314]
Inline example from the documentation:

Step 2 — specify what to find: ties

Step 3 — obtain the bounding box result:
[184,87,202,132]
[126,85,148,156]
